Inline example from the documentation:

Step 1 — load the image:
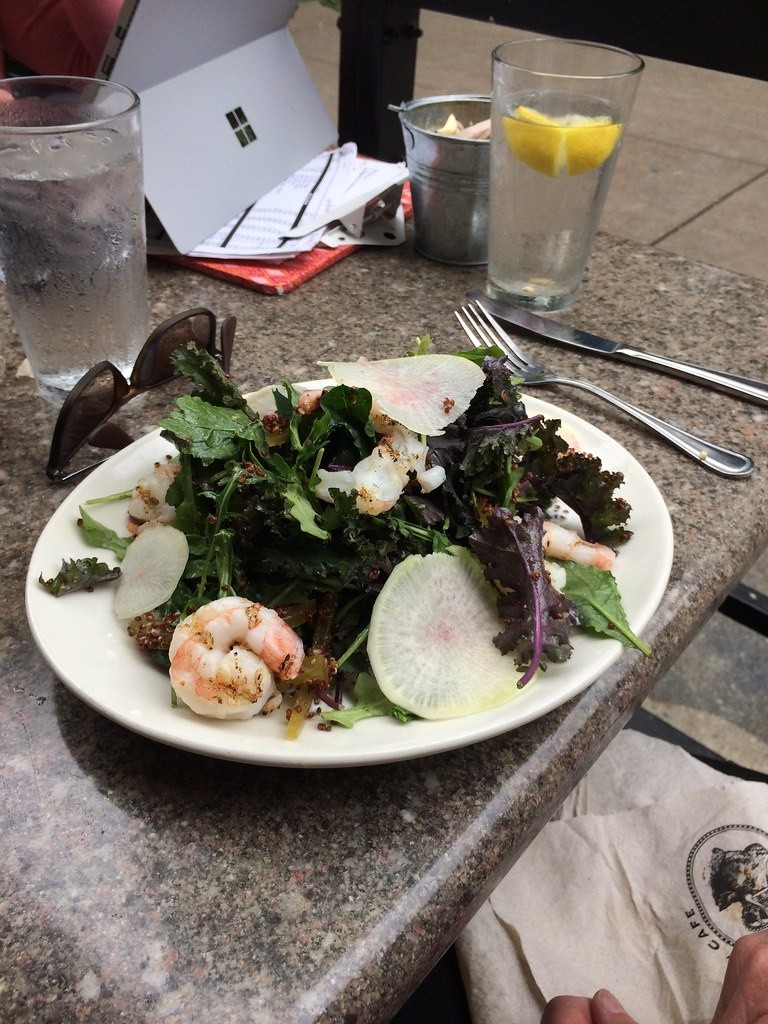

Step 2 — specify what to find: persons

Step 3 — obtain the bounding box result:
[1,1,124,230]
[390,706,767,1024]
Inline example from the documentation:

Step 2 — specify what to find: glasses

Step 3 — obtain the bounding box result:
[44,307,236,484]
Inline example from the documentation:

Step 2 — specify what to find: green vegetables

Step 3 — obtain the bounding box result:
[37,334,652,729]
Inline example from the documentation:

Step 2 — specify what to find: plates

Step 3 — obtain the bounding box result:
[26,380,675,766]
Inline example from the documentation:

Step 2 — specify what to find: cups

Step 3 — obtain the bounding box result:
[400,94,500,265]
[489,40,646,309]
[0,76,148,408]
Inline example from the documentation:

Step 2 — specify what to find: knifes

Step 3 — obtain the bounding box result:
[465,290,768,405]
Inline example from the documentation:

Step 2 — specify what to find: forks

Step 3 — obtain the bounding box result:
[454,300,755,477]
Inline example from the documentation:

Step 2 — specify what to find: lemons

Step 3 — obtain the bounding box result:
[504,106,623,175]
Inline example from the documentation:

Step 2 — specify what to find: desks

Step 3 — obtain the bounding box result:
[0,226,768,1024]
